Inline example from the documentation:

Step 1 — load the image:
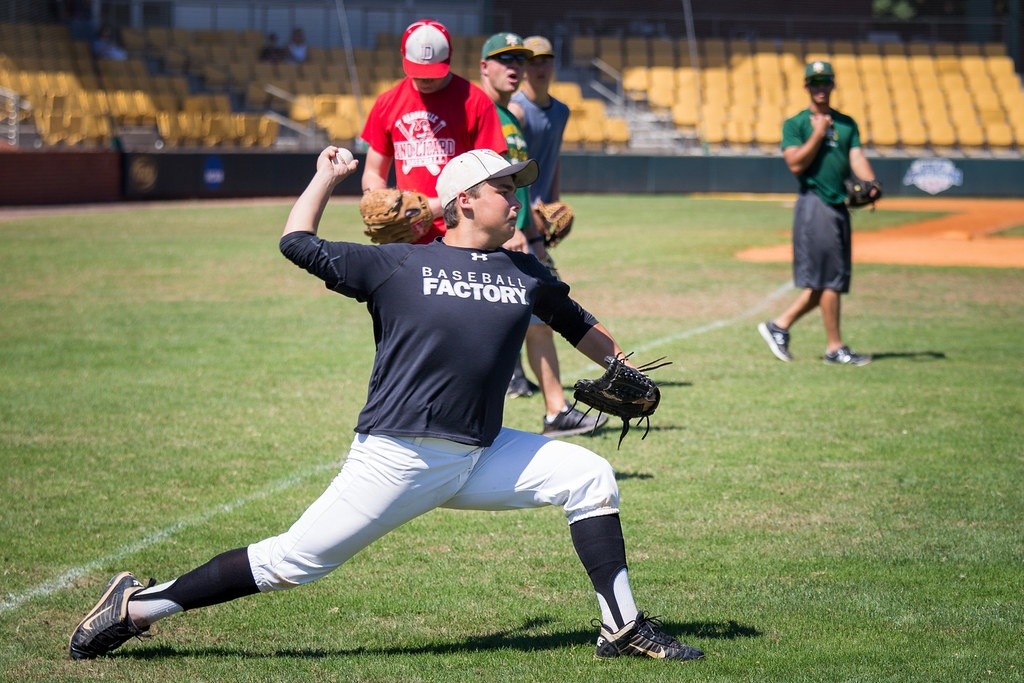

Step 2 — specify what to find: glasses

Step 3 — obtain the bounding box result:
[809,79,832,88]
[485,53,526,67]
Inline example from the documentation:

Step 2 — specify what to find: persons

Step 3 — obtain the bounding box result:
[67,148,705,662]
[360,18,609,440]
[93,24,127,62]
[758,60,882,367]
[261,28,311,64]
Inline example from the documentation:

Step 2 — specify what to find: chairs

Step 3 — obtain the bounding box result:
[0,20,1023,153]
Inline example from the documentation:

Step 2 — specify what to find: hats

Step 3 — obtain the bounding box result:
[482,32,534,60]
[805,62,835,80]
[401,19,451,79]
[435,148,540,210]
[523,35,555,59]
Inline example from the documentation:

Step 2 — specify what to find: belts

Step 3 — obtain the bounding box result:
[395,435,478,457]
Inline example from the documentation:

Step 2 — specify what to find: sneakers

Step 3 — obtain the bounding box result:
[68,571,155,660]
[541,399,609,437]
[508,377,533,399]
[590,610,706,662]
[757,320,793,362]
[823,345,872,367]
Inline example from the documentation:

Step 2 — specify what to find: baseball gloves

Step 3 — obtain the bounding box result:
[564,350,675,452]
[358,185,434,245]
[534,198,575,250]
[845,178,884,207]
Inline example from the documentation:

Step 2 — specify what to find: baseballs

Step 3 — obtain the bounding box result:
[332,147,354,167]
